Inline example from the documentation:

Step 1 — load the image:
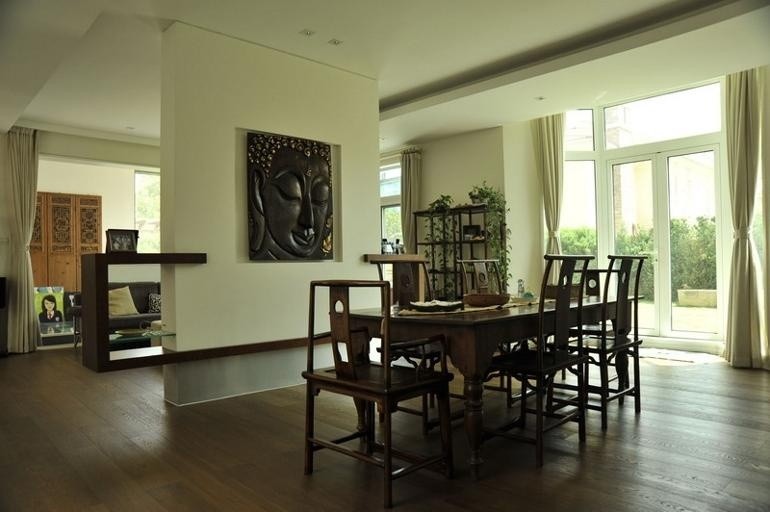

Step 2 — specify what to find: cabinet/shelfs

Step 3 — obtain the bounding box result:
[412,203,503,300]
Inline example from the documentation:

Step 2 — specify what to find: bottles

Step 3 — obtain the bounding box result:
[518,279,526,297]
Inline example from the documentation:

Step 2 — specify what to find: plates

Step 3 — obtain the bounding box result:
[463,294,510,307]
[115,329,147,338]
[510,297,537,302]
[410,299,463,312]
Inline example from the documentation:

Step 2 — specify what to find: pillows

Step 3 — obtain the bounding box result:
[107,285,139,316]
[148,293,161,313]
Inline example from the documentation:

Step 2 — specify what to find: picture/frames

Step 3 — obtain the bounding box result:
[105,229,139,253]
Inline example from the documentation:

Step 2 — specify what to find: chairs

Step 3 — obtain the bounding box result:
[561,269,619,380]
[301,280,454,512]
[456,258,505,388]
[567,255,647,429]
[366,253,441,408]
[489,254,596,467]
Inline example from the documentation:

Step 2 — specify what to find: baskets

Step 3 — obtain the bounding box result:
[462,292,512,308]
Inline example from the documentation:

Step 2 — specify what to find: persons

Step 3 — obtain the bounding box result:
[247,132,334,260]
[38,295,64,323]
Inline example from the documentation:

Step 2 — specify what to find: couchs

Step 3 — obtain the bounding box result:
[108,281,160,332]
